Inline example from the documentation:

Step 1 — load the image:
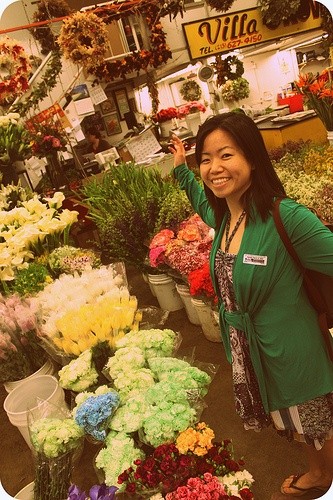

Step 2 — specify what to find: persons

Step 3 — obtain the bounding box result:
[86,128,110,154]
[168,112,333,496]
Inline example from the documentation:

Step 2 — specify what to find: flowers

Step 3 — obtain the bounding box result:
[1,14,333,500]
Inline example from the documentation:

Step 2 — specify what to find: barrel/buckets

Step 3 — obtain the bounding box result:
[4,358,55,397]
[148,273,183,313]
[3,375,69,456]
[142,273,156,300]
[14,480,73,500]
[174,283,200,325]
[190,297,224,343]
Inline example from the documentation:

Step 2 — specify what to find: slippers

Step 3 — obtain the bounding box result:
[280,472,329,497]
